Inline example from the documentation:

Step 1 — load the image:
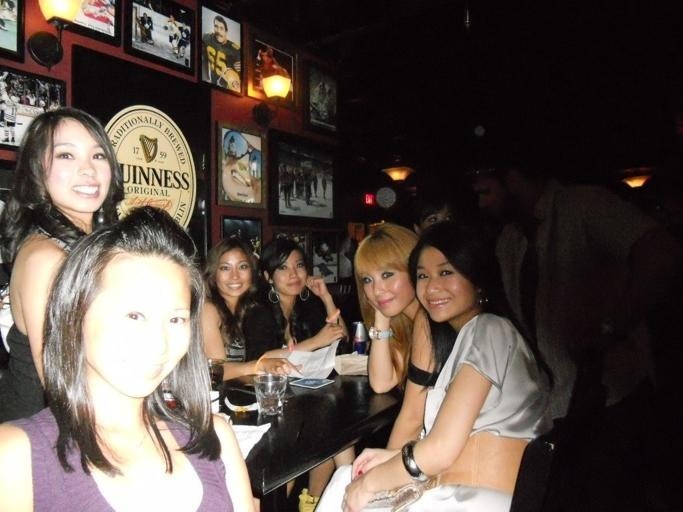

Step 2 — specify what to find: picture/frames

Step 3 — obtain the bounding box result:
[197,1,245,97]
[0,0,26,64]
[220,215,263,289]
[268,127,346,228]
[272,230,310,281]
[64,0,122,47]
[0,160,18,286]
[297,48,345,132]
[0,66,66,152]
[124,0,195,77]
[245,24,298,112]
[217,120,267,210]
[309,229,339,284]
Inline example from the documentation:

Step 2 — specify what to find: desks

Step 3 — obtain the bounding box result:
[202,366,402,512]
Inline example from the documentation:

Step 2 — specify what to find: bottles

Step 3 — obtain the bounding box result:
[352,321,368,355]
[207,358,225,395]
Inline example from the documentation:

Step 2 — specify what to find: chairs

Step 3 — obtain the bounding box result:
[510,426,561,512]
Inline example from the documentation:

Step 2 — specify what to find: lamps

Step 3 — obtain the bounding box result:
[381,153,415,182]
[616,164,652,190]
[28,0,80,70]
[252,66,292,124]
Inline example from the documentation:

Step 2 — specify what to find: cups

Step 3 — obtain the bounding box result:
[254,374,288,416]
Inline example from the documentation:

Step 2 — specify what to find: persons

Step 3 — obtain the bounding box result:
[0,207,234,511]
[0,108,123,424]
[2,71,61,144]
[201,139,683,512]
[202,15,241,93]
[257,46,289,78]
[137,10,191,59]
